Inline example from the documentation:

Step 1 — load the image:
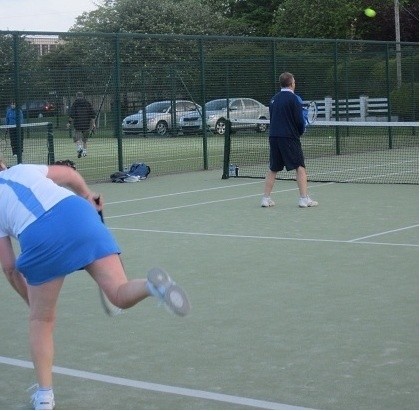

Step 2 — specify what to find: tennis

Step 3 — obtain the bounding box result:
[364,8,376,17]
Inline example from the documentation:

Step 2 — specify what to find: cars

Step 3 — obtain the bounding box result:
[121,101,202,138]
[21,99,57,119]
[179,98,270,136]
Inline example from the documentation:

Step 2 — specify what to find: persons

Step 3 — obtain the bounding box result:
[4,101,24,159]
[261,72,318,207]
[0,161,192,410]
[68,91,96,159]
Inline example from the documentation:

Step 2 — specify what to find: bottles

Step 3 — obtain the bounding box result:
[235,164,240,177]
[230,164,235,177]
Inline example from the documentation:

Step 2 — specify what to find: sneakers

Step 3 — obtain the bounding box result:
[298,197,318,207]
[30,390,55,410]
[77,148,83,158]
[147,266,191,318]
[83,148,87,157]
[261,198,275,207]
